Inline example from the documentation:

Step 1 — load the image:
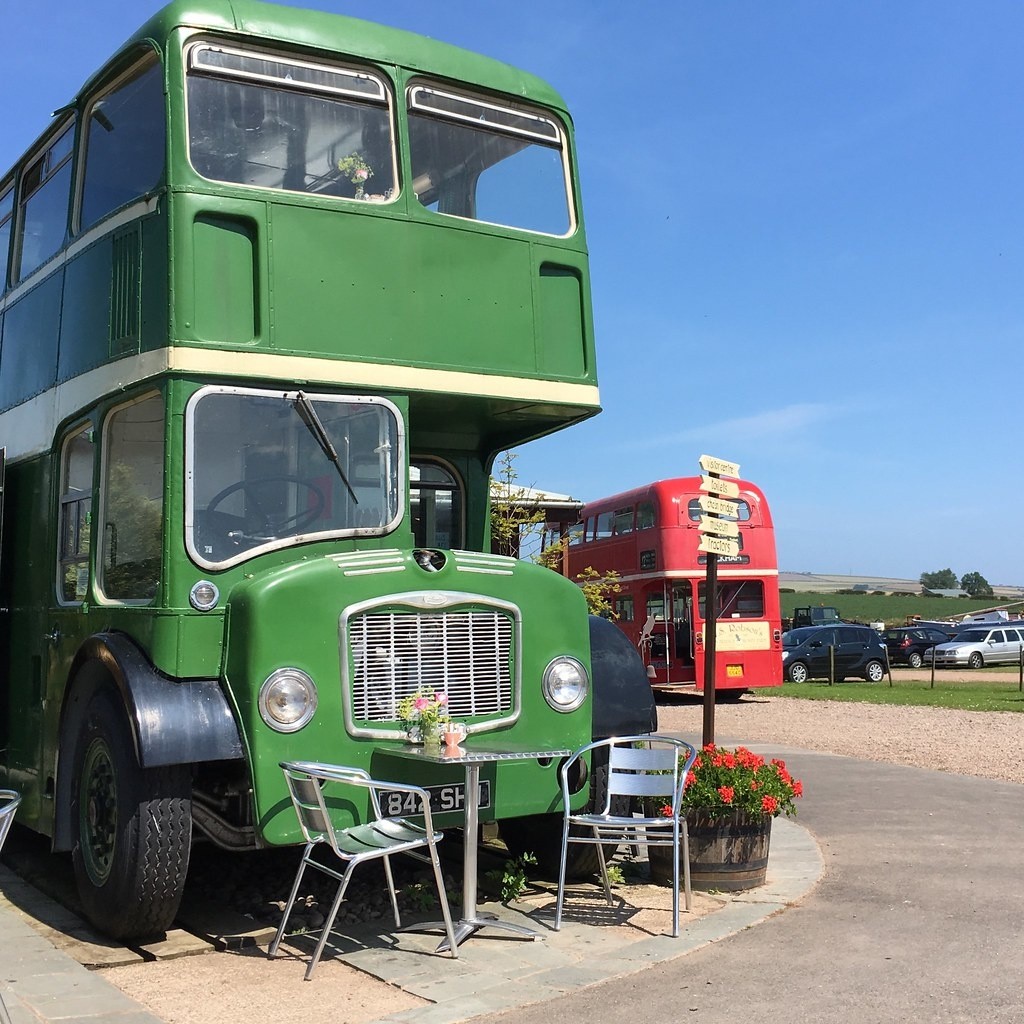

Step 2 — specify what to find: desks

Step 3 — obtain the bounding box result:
[373,745,571,953]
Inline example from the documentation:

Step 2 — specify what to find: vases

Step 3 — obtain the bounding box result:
[355,181,369,201]
[642,800,772,891]
[424,723,440,757]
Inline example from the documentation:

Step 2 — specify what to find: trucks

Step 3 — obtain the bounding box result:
[781,607,845,631]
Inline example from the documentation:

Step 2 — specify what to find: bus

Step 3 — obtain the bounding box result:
[541,476,784,700]
[0,0,658,945]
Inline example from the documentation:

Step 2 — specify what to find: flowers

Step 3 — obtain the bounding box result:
[637,743,804,820]
[339,151,374,186]
[397,686,450,741]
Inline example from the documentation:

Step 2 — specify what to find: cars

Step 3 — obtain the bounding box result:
[923,625,1024,669]
[778,625,889,683]
[879,627,956,669]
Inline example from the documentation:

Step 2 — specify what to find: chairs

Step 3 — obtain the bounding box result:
[264,759,458,981]
[0,790,22,1024]
[552,734,698,939]
[196,510,251,562]
